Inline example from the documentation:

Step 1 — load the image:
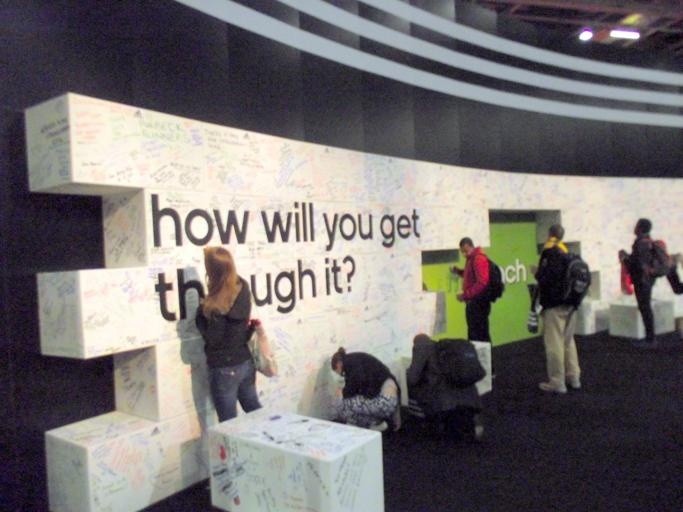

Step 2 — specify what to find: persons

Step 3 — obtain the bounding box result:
[617,216,658,349]
[329,347,403,434]
[526,245,582,397]
[543,222,569,255]
[193,244,263,424]
[406,332,483,439]
[652,239,682,295]
[448,236,497,379]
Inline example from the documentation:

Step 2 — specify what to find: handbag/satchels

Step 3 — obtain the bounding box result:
[620,257,635,295]
[245,319,278,378]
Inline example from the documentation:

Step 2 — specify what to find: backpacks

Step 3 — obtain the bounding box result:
[637,238,669,277]
[423,337,486,389]
[470,253,505,303]
[550,245,592,306]
